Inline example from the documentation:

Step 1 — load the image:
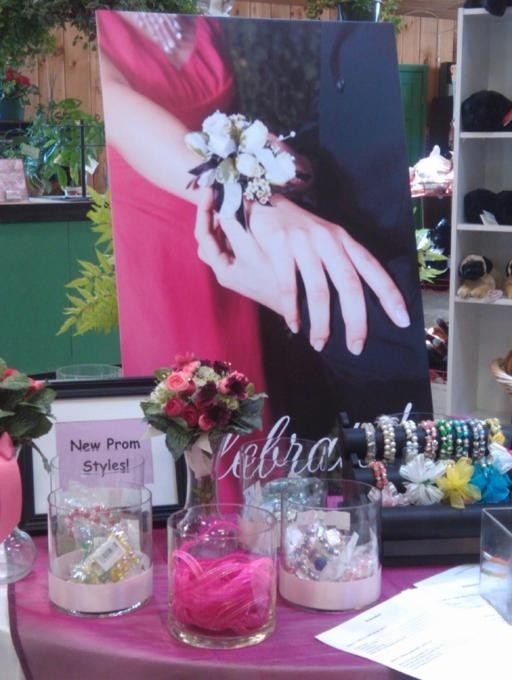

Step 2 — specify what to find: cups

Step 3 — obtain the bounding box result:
[165,503,281,651]
[278,478,384,611]
[47,479,155,620]
[237,437,330,556]
[55,364,122,381]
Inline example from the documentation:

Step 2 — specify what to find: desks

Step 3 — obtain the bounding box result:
[0,535,476,679]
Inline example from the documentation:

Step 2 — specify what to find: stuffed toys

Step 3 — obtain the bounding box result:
[455,254,496,300]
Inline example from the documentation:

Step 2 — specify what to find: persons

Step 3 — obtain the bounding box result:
[97,7,417,419]
[192,21,433,428]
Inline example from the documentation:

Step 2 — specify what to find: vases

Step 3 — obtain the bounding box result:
[0,431,23,545]
[168,430,232,530]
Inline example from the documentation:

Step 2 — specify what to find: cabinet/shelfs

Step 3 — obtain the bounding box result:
[446,5,512,418]
[1,206,131,384]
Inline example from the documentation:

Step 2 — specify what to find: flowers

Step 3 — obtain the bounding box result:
[138,352,269,514]
[1,359,56,451]
[182,111,295,205]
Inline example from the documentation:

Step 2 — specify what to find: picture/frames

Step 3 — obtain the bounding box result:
[21,374,187,537]
